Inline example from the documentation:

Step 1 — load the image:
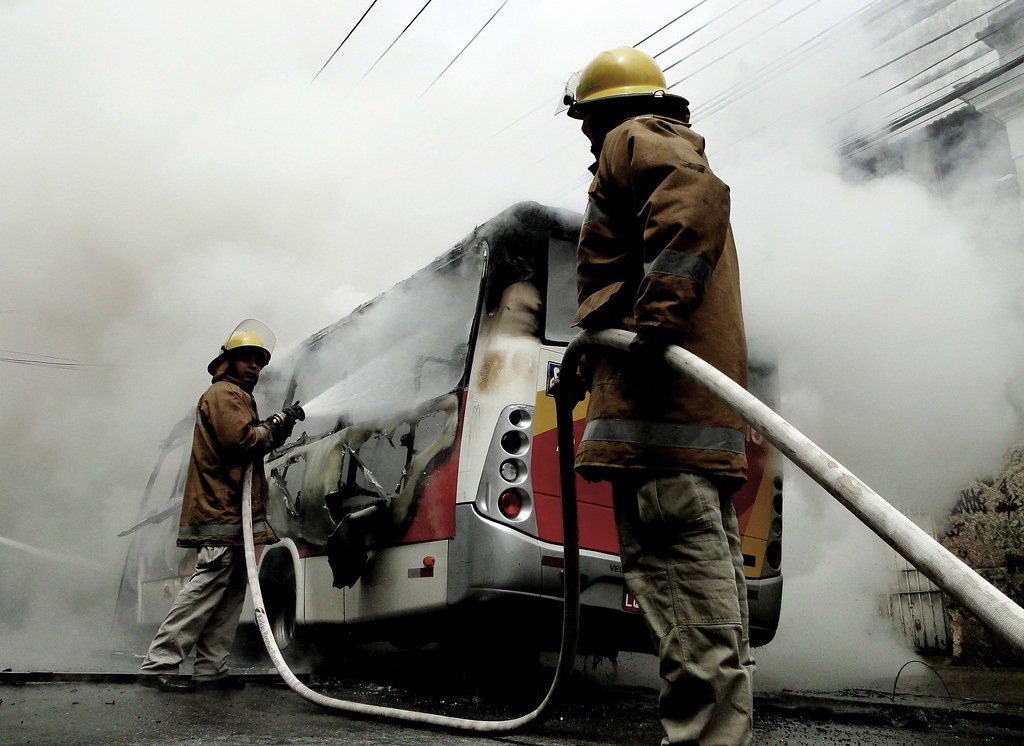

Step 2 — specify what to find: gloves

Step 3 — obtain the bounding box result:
[628,330,683,397]
[282,400,306,425]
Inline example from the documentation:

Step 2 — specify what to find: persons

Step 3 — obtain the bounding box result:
[554,47,756,746]
[138,319,306,694]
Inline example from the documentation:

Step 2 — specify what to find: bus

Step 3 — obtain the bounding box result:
[117,203,783,676]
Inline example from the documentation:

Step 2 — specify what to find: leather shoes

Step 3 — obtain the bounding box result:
[138,674,196,692]
[190,675,246,691]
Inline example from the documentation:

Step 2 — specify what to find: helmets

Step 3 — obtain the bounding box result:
[208,331,272,376]
[567,46,690,119]
